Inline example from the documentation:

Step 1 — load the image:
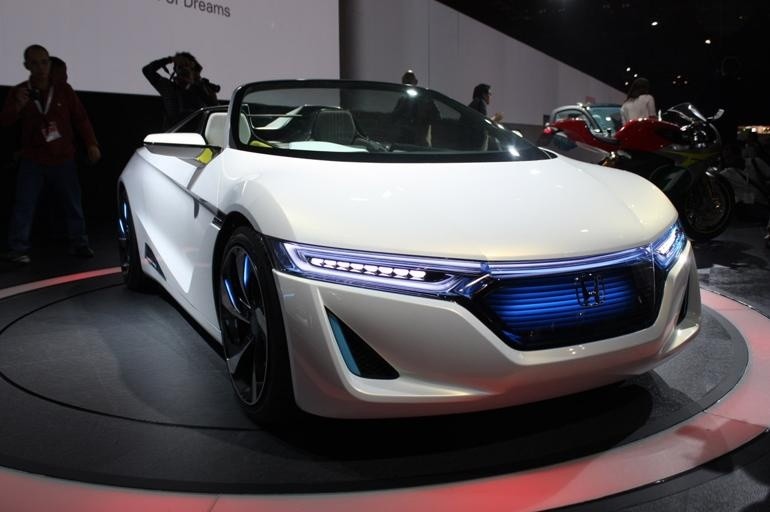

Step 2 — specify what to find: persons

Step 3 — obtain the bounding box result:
[140,51,217,132]
[391,69,442,148]
[455,81,505,151]
[713,144,769,226]
[619,75,659,126]
[0,43,103,264]
[48,56,70,88]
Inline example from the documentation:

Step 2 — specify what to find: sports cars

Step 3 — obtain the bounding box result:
[113,79,703,422]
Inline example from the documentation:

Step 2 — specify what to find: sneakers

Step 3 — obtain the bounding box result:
[72,243,95,259]
[11,254,31,264]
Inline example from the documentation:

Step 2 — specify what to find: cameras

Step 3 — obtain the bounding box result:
[191,77,220,105]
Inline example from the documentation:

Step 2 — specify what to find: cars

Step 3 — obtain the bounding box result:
[545,104,624,137]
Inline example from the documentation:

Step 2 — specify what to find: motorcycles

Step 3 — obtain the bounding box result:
[720,127,770,207]
[535,105,736,242]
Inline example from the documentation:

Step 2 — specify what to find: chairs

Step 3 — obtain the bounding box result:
[312,109,379,153]
[587,114,601,132]
[204,110,275,150]
